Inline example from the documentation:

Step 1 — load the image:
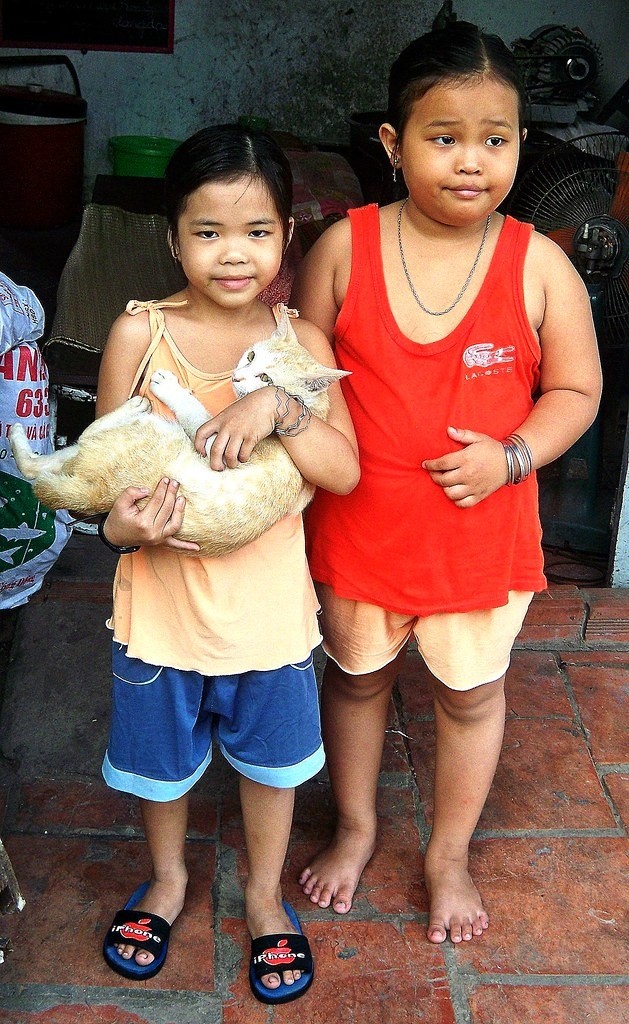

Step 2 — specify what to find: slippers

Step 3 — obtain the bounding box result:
[102,876,172,978]
[249,896,315,1006]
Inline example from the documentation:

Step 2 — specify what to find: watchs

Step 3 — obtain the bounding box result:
[98,513,142,554]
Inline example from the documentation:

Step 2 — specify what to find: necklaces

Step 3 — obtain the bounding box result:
[398,198,492,316]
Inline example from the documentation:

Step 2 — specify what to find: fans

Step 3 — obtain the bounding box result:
[501,129,629,556]
[518,21,603,108]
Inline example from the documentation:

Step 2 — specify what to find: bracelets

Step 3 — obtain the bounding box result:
[500,434,535,487]
[274,385,311,437]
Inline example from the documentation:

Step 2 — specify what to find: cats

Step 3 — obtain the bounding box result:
[7,305,353,559]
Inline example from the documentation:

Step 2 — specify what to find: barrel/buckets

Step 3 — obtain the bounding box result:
[107,135,185,178]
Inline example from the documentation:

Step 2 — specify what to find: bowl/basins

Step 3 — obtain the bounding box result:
[239,116,269,130]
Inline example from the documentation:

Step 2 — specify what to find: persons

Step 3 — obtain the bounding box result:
[285,24,607,948]
[97,120,363,1003]
